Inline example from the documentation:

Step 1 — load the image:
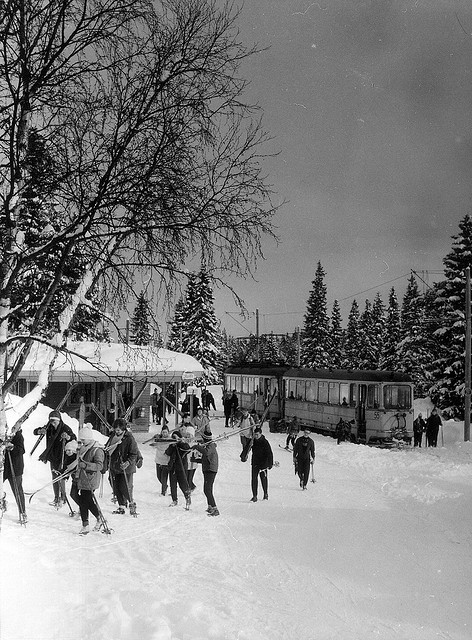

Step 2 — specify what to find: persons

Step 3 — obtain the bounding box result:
[33,411,77,505]
[152,425,172,497]
[241,428,273,502]
[201,389,206,409]
[83,442,87,443]
[206,390,216,411]
[180,413,193,427]
[74,423,104,534]
[284,416,300,449]
[293,428,315,491]
[104,419,140,514]
[150,388,160,422]
[183,427,198,490]
[0,428,26,521]
[156,392,169,425]
[52,440,80,506]
[231,389,238,414]
[165,430,191,507]
[192,408,210,436]
[413,413,426,447]
[239,409,257,459]
[425,409,442,447]
[224,400,231,427]
[189,431,219,516]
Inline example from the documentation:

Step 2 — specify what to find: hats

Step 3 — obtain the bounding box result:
[202,431,212,440]
[50,411,61,420]
[65,440,78,451]
[79,423,93,439]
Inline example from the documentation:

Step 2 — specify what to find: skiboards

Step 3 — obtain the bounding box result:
[23,468,77,504]
[2,401,39,449]
[294,460,298,476]
[279,444,293,453]
[161,395,206,439]
[240,388,277,459]
[89,402,115,431]
[149,438,204,447]
[123,378,150,420]
[142,424,183,445]
[179,423,261,459]
[29,383,78,456]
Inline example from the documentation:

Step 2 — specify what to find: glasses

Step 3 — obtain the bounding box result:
[304,431,310,434]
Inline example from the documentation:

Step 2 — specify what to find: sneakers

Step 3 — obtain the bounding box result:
[303,486,307,490]
[300,481,303,487]
[206,508,213,513]
[170,501,177,506]
[208,509,219,517]
[58,500,66,505]
[264,495,268,501]
[186,496,191,504]
[250,497,257,502]
[130,508,135,514]
[113,508,124,514]
[94,519,102,532]
[49,499,57,505]
[79,525,90,534]
[284,446,289,449]
